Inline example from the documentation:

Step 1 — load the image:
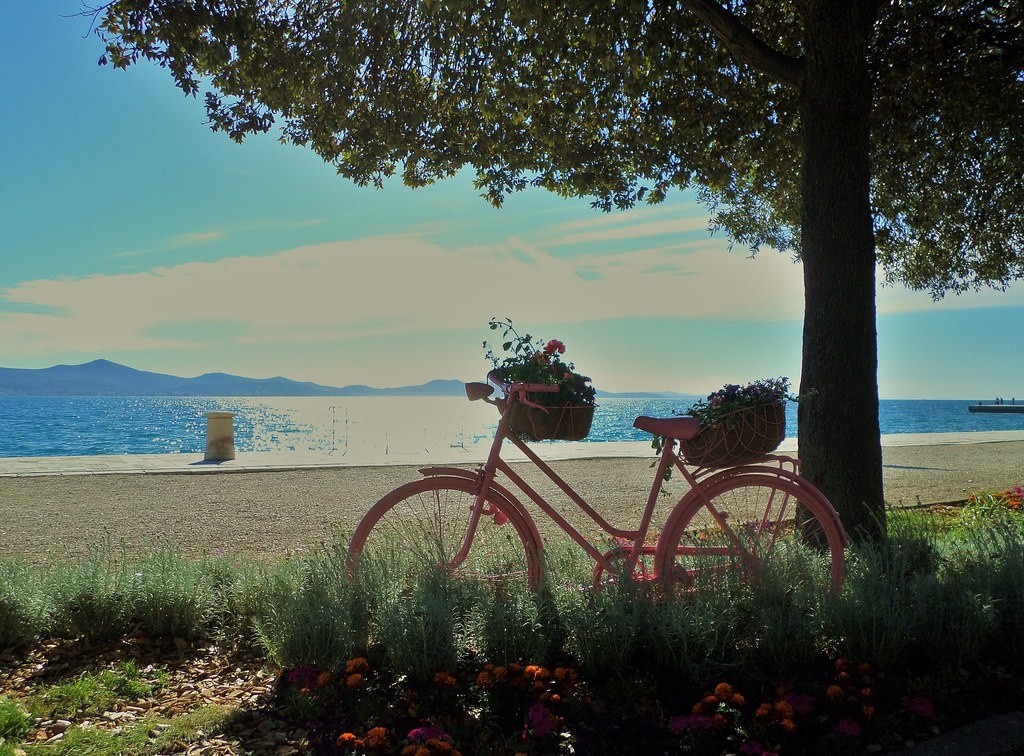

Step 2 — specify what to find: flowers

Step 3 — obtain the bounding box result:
[481,317,598,407]
[651,375,821,496]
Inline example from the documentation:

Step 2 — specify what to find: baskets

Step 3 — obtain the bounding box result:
[501,399,596,443]
[680,400,786,469]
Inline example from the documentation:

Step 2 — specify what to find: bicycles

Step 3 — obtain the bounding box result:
[346,369,849,640]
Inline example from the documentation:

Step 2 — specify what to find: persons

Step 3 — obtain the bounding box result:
[1012,397,1015,404]
[996,397,1003,404]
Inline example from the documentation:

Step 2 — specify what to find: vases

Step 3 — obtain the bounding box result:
[679,401,787,469]
[503,394,599,442]
